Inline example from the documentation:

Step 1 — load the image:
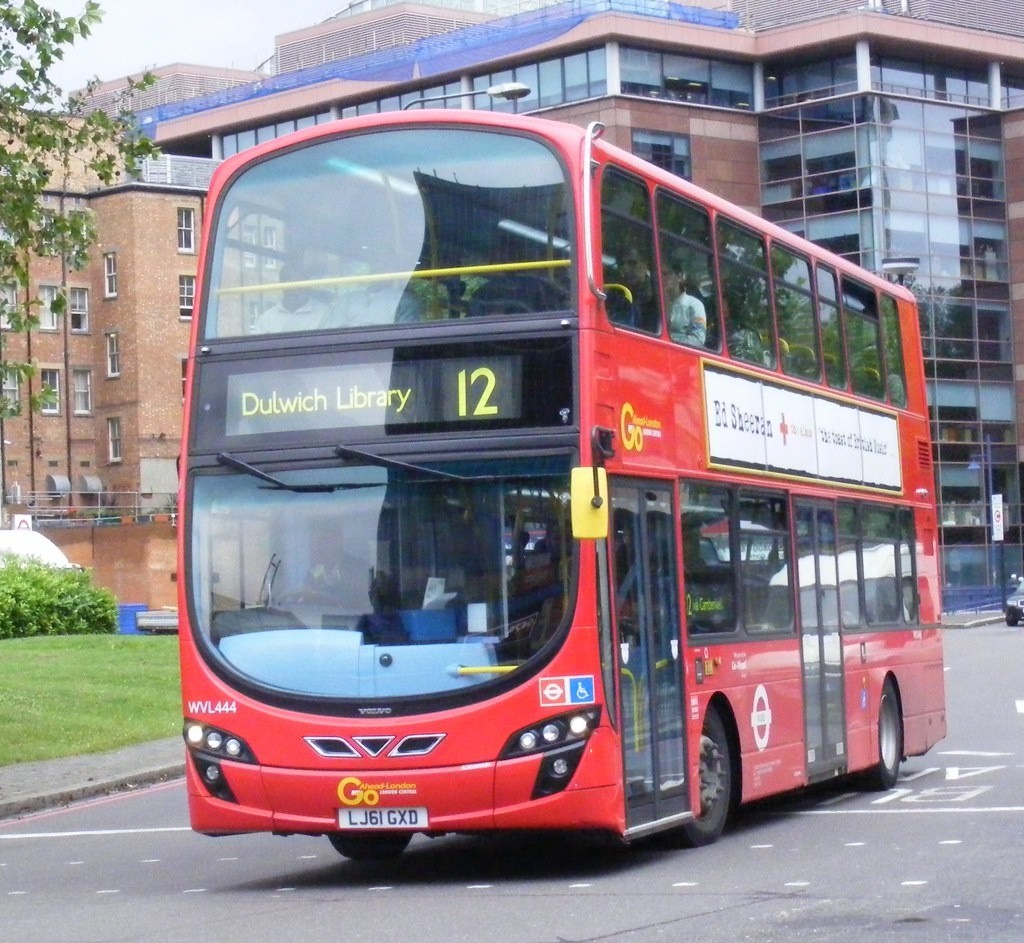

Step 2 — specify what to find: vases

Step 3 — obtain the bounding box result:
[66,516,78,526]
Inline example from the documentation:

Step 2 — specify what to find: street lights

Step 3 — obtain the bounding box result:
[401,82,532,110]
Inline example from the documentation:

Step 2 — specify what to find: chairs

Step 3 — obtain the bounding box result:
[682,576,919,635]
[312,273,908,409]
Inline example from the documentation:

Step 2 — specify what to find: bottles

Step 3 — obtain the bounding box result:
[696,658,704,684]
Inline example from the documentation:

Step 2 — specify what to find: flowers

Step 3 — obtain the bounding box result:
[67,508,78,516]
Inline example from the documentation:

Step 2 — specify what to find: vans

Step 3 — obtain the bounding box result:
[761,538,930,710]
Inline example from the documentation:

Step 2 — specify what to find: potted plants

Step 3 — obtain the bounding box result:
[79,507,169,526]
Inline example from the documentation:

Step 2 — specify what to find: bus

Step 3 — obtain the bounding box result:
[173,109,949,873]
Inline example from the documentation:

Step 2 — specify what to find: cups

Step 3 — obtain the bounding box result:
[705,660,713,675]
[714,657,721,666]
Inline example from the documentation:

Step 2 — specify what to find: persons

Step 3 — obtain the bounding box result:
[253,263,332,332]
[605,244,765,365]
[946,494,983,526]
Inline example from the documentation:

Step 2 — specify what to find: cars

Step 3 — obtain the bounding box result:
[1003,580,1024,627]
[0,528,82,572]
